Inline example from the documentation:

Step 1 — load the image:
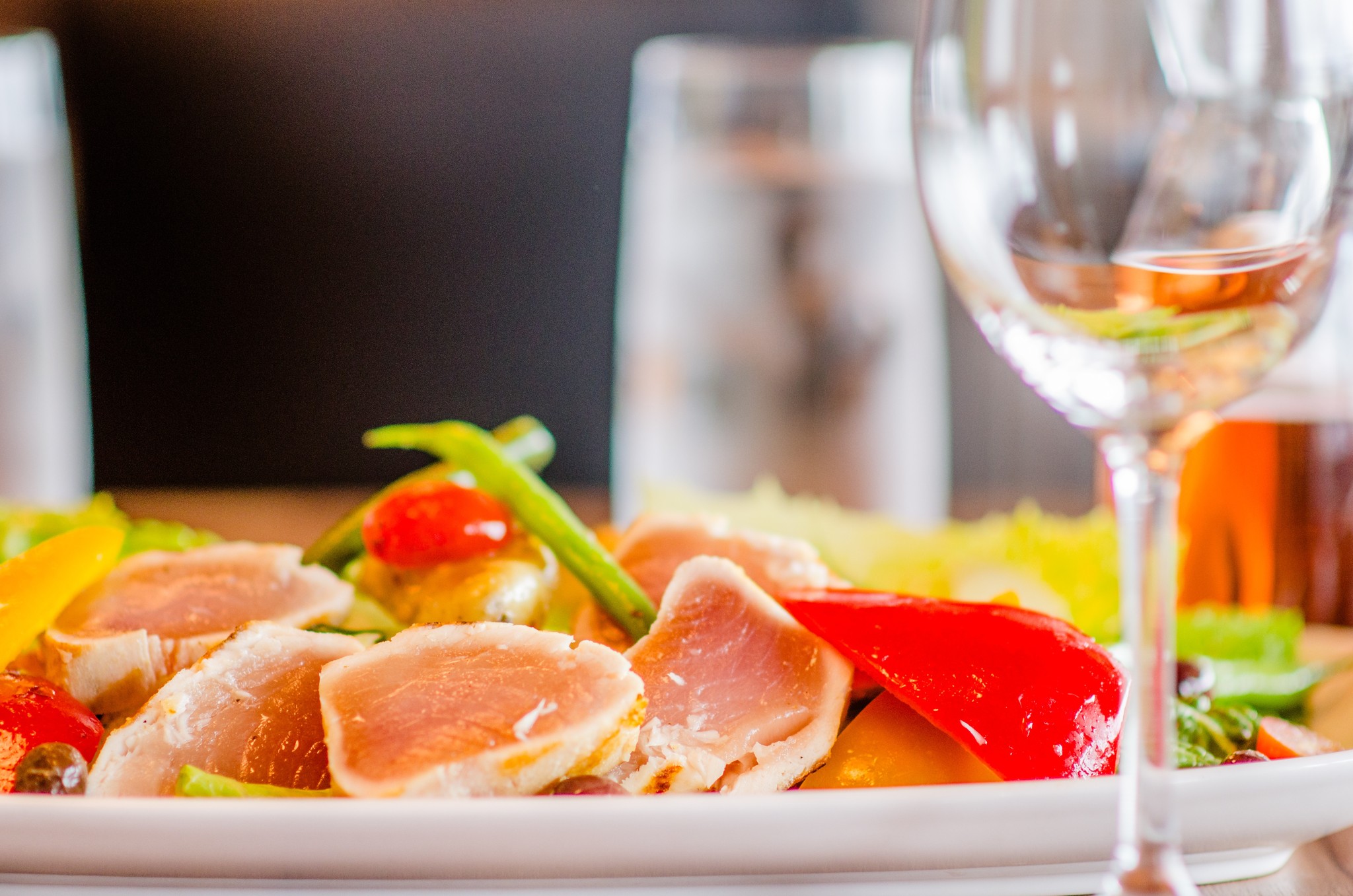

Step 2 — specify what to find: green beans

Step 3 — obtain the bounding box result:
[304,413,664,637]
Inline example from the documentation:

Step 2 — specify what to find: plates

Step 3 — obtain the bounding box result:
[2,653,1353,896]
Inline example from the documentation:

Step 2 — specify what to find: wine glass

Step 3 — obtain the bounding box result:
[920,0,1350,895]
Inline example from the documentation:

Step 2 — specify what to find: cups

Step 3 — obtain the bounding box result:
[611,34,953,545]
[1094,242,1350,641]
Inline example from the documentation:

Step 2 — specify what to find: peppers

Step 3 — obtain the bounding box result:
[0,489,1350,801]
